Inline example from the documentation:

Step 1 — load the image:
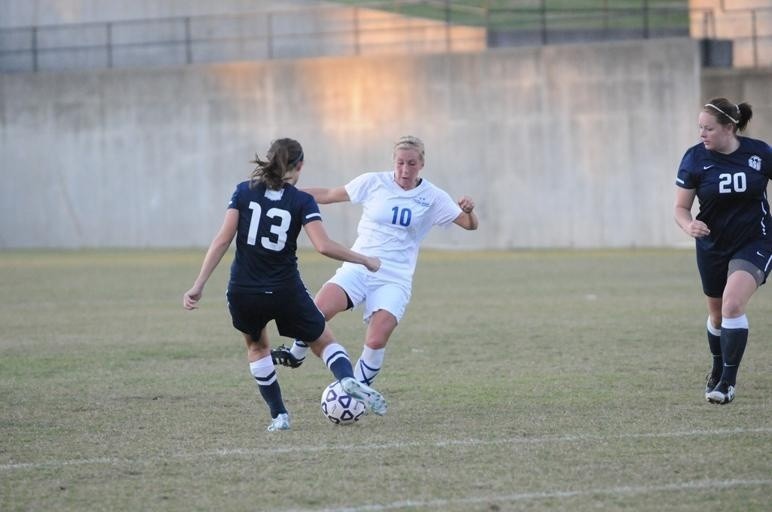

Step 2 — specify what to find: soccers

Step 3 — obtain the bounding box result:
[321,379,367,424]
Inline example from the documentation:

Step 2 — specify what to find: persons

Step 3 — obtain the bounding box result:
[182,138,390,435]
[672,94,772,404]
[269,134,479,389]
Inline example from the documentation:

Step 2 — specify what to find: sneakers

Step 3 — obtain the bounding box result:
[267,412,289,432]
[341,377,389,416]
[705,375,736,404]
[270,345,306,368]
